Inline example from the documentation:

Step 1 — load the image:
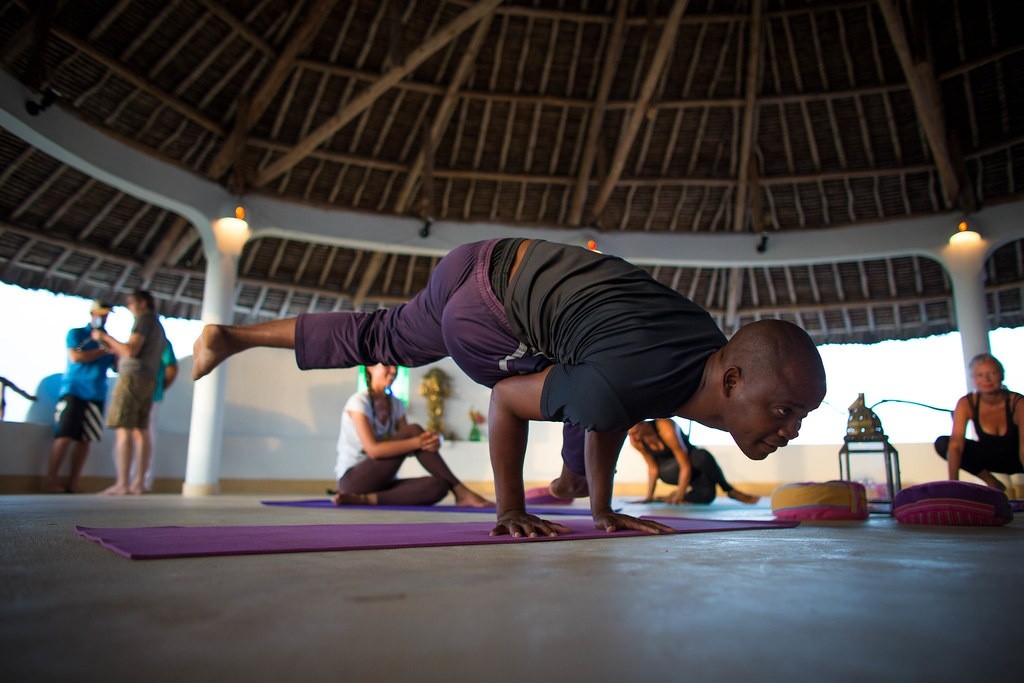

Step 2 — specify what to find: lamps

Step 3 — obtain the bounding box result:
[947,218,982,245]
[26,88,62,116]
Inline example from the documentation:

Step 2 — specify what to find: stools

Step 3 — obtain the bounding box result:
[837,435,901,504]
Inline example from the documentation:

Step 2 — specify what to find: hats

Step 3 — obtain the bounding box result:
[91,302,115,313]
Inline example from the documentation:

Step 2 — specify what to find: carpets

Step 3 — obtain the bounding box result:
[260,499,623,514]
[73,515,802,560]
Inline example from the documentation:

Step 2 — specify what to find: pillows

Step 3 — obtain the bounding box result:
[770,480,869,521]
[893,479,1015,526]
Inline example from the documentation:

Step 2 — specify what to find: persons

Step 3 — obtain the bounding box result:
[115,338,177,492]
[192,237,827,538]
[41,300,116,493]
[332,363,494,508]
[935,353,1024,491]
[91,291,166,495]
[627,419,759,507]
